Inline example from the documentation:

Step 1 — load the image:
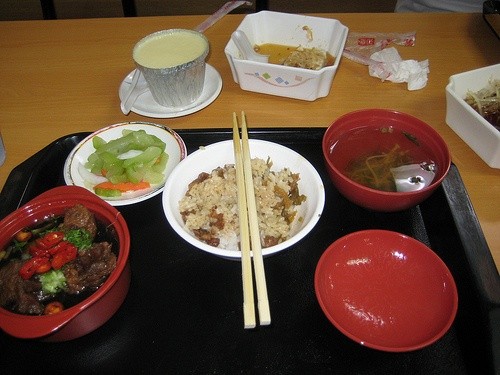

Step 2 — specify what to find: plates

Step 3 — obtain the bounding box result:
[118,62,223,118]
[316,230,457,351]
[64,121,188,206]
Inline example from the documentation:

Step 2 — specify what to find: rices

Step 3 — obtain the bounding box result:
[177,157,294,250]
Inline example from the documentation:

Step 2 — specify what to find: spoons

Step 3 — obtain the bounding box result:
[232,30,270,63]
[120,70,150,115]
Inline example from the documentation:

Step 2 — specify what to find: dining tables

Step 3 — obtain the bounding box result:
[0,12,500,274]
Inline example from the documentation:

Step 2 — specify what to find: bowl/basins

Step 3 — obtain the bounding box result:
[445,63,500,169]
[224,10,349,101]
[132,29,209,107]
[0,187,131,339]
[323,110,452,211]
[162,141,325,258]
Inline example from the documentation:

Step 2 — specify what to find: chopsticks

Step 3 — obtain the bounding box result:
[232,110,272,329]
